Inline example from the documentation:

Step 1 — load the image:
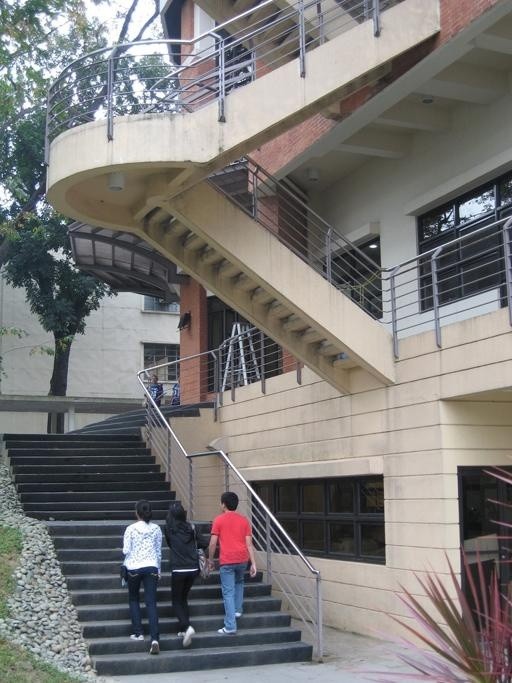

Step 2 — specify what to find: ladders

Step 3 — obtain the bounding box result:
[221,321,260,392]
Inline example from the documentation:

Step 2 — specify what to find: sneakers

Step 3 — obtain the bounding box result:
[235,612,242,618]
[150,640,160,655]
[217,626,237,634]
[178,625,195,647]
[130,634,144,641]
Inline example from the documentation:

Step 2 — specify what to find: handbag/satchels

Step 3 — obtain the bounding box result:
[196,548,209,580]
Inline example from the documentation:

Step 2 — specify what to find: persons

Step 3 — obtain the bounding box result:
[173,377,182,404]
[164,505,203,647]
[141,376,164,407]
[123,498,162,654]
[209,493,258,633]
